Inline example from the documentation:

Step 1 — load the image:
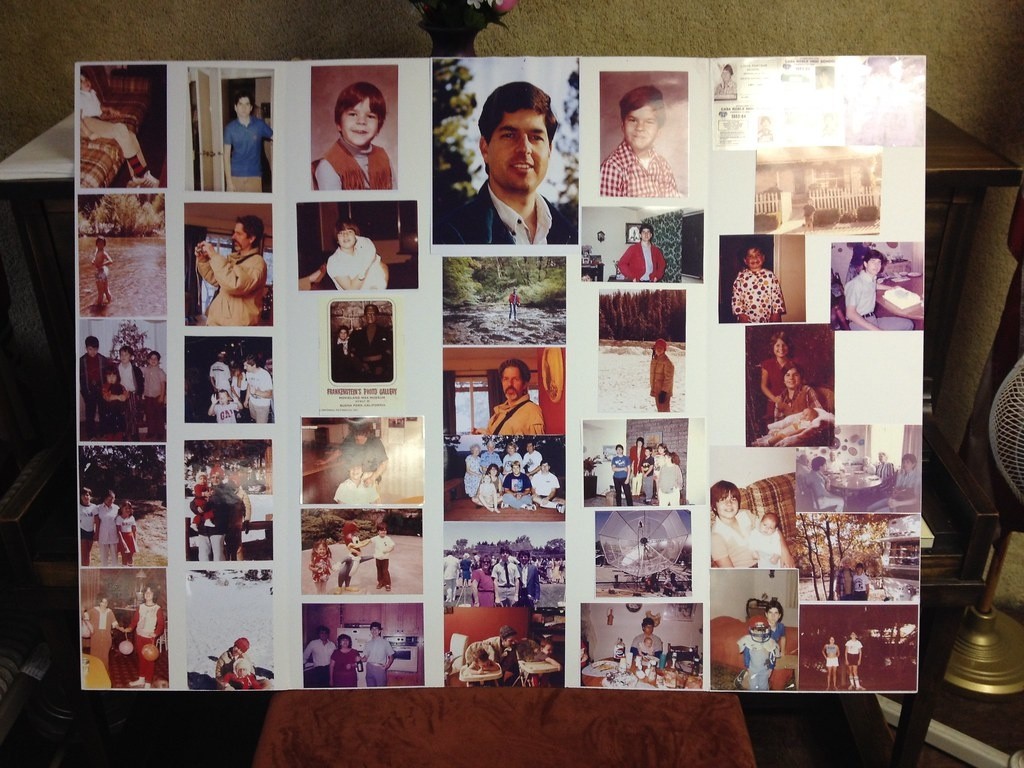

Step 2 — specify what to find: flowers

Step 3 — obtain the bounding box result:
[409,0,518,30]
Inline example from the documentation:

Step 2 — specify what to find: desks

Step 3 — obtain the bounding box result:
[250,688,756,768]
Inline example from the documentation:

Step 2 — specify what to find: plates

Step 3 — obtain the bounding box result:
[908,272,921,277]
[642,654,658,667]
[582,660,617,677]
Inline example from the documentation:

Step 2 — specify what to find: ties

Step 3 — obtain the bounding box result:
[504,563,510,588]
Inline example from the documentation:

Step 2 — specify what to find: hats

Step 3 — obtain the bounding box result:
[235,637,249,653]
[464,553,469,558]
[656,339,667,350]
[194,471,208,482]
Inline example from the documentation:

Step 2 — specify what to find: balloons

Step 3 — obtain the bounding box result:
[143,644,159,662]
[119,640,133,655]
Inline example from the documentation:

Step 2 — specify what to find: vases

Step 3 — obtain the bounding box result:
[417,20,486,56]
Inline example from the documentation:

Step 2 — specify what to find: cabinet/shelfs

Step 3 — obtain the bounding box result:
[905,189,987,376]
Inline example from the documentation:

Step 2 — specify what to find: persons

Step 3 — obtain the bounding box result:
[77,64,919,692]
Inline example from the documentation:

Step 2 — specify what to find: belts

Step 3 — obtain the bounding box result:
[848,312,873,322]
[632,280,650,282]
[498,585,515,587]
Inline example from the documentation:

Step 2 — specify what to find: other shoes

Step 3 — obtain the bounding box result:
[556,503,564,513]
[204,520,216,527]
[848,685,866,691]
[525,504,536,511]
[377,583,383,589]
[385,584,391,590]
[190,524,199,531]
[489,508,501,513]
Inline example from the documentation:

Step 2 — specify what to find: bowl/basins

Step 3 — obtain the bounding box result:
[680,661,692,672]
[899,271,907,276]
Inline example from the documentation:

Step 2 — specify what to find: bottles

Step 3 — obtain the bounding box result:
[356,655,363,672]
[692,659,699,676]
[671,652,677,670]
[648,660,657,682]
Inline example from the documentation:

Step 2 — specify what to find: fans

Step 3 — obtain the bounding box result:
[989,356,1024,506]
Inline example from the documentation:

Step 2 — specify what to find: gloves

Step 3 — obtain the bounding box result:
[243,521,249,534]
[658,391,666,404]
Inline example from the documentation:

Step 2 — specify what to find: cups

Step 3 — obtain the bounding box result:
[625,652,633,668]
[659,654,666,668]
[618,657,625,671]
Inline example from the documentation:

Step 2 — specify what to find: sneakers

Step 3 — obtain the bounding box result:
[129,170,160,188]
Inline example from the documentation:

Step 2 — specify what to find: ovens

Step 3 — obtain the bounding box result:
[383,637,418,673]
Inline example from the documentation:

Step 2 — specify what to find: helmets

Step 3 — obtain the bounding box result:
[747,614,770,634]
[210,466,225,479]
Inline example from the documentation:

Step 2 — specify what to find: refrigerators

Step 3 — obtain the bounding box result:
[336,623,373,687]
